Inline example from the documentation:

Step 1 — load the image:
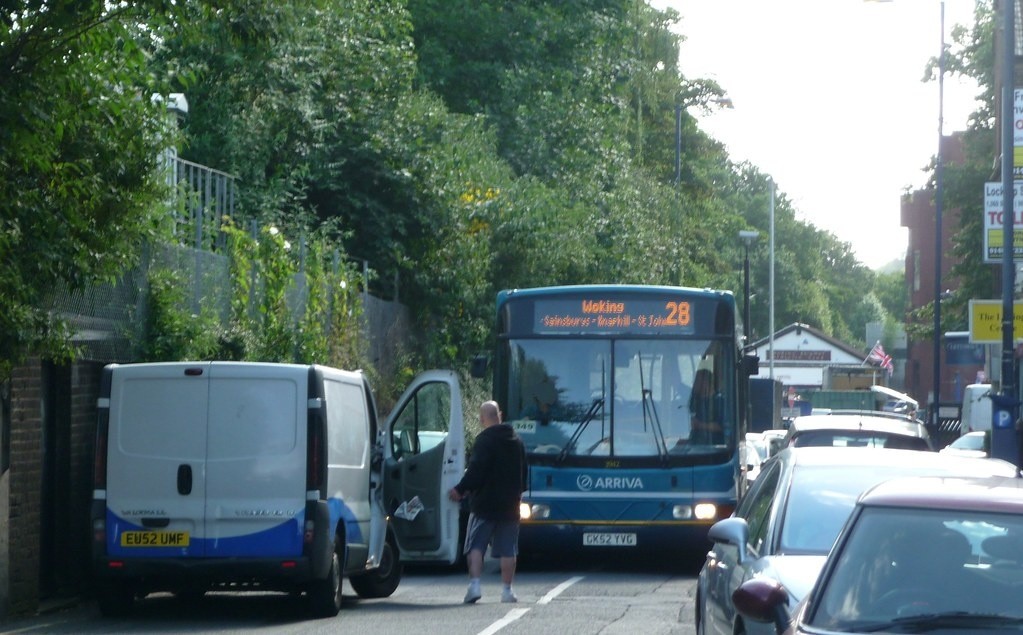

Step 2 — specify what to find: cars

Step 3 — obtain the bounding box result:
[727,474,1023,635]
[377,426,445,455]
[690,455,1023,635]
[741,428,879,504]
[777,414,936,457]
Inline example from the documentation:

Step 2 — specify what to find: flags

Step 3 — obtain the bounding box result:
[868,341,894,376]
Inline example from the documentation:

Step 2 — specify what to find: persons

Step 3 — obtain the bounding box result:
[450,401,526,603]
[676,369,729,445]
[521,387,574,452]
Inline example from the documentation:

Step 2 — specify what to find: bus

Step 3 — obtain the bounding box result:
[473,283,760,571]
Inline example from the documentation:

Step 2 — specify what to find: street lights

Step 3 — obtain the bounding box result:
[675,96,731,284]
[740,228,761,346]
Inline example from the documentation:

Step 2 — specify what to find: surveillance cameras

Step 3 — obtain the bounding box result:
[739,230,760,240]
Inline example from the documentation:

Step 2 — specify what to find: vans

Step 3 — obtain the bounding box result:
[98,358,468,613]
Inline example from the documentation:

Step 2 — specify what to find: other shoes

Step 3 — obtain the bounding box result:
[464,585,481,603]
[500,592,517,603]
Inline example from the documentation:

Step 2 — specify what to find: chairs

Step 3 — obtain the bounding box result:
[941,529,1023,586]
